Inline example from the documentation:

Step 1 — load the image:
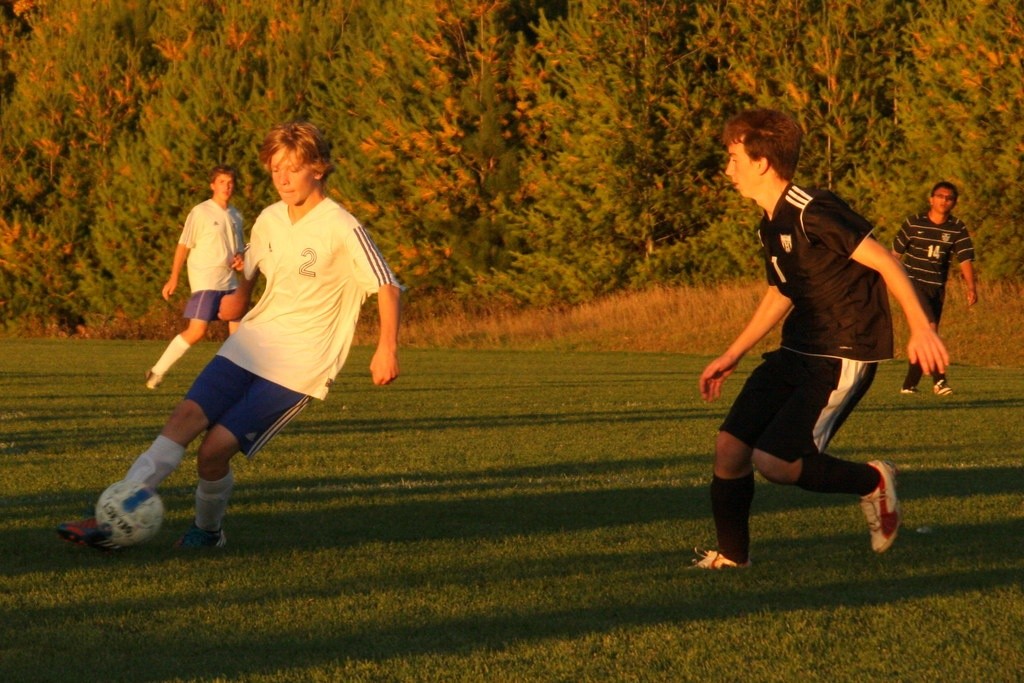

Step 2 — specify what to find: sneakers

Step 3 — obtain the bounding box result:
[901,387,919,394]
[861,460,901,551]
[689,546,748,568]
[933,380,953,395]
[174,522,227,548]
[145,369,161,389]
[55,517,122,552]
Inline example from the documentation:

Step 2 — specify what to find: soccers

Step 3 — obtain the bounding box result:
[95,477,165,547]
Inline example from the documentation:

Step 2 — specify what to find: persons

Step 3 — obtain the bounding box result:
[55,120,406,550]
[688,107,948,570]
[889,182,977,396]
[143,165,246,388]
